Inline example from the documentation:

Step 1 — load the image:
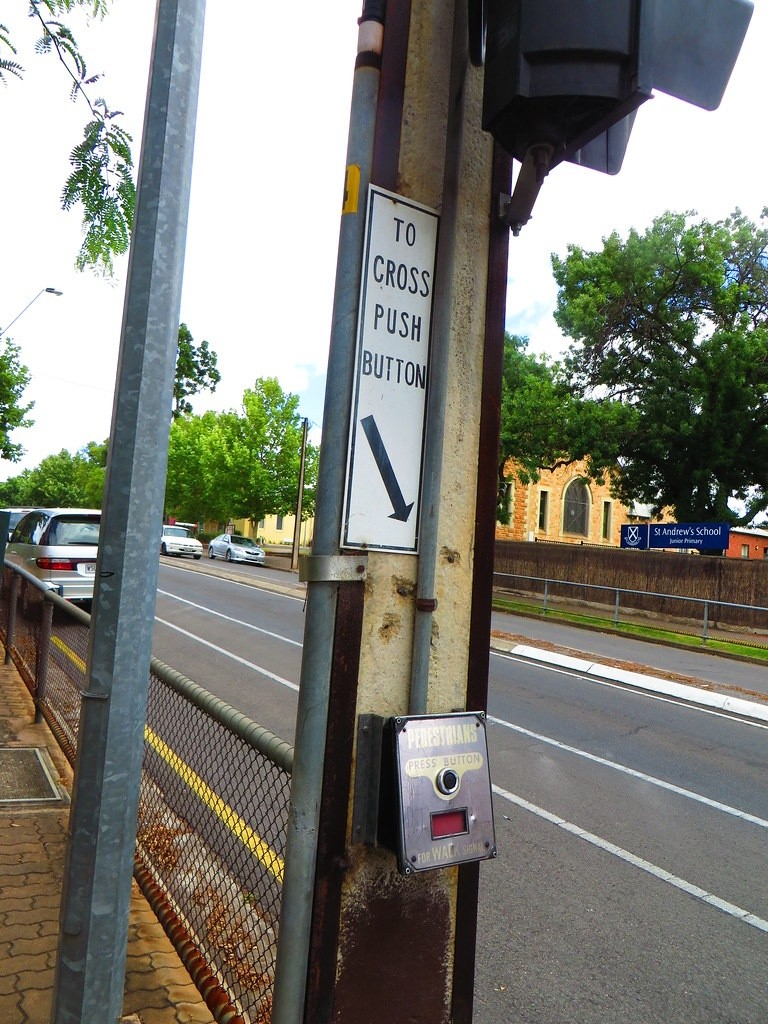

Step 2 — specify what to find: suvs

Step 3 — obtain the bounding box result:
[0,506,102,618]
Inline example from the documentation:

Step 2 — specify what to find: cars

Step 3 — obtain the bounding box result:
[208,534,266,567]
[160,525,204,559]
